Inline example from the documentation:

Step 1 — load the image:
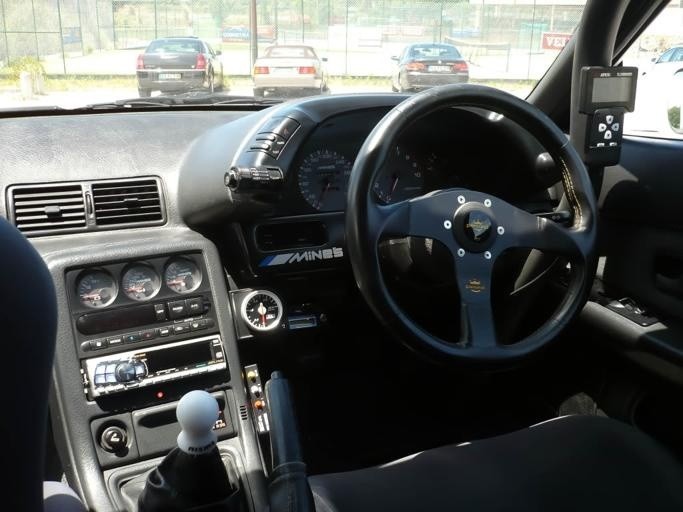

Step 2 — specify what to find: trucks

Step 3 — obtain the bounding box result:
[220,14,313,46]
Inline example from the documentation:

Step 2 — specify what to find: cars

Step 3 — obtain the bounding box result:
[133,34,225,97]
[388,43,469,93]
[252,43,329,101]
[635,43,683,75]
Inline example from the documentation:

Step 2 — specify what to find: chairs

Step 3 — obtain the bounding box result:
[307,413,683,510]
